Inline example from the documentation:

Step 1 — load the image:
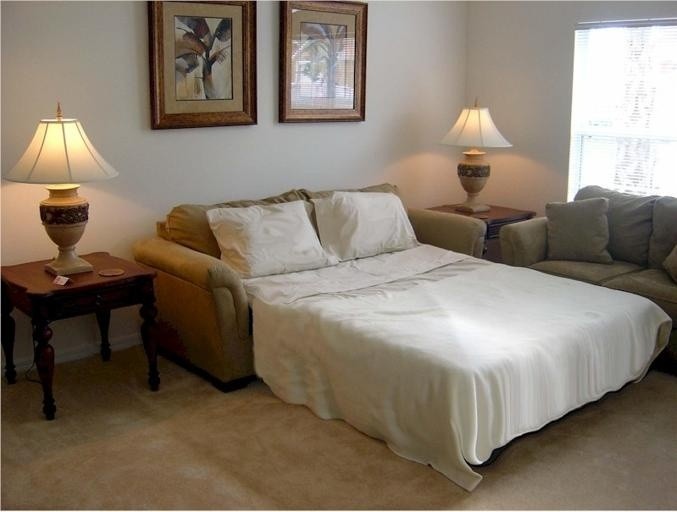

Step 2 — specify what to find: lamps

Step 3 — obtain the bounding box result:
[439,97,519,219]
[5,94,126,284]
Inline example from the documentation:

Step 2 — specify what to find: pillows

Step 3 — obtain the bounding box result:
[308,187,421,266]
[660,243,677,283]
[541,196,617,266]
[205,197,339,283]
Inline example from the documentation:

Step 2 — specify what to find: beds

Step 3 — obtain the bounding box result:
[206,180,672,495]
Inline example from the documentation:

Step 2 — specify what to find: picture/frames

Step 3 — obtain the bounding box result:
[276,0,373,126]
[144,0,260,132]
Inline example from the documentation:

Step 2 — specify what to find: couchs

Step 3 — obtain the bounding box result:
[133,183,488,395]
[498,183,676,362]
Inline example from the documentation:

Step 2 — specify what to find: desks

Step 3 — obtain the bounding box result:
[426,202,538,245]
[1,246,171,420]
